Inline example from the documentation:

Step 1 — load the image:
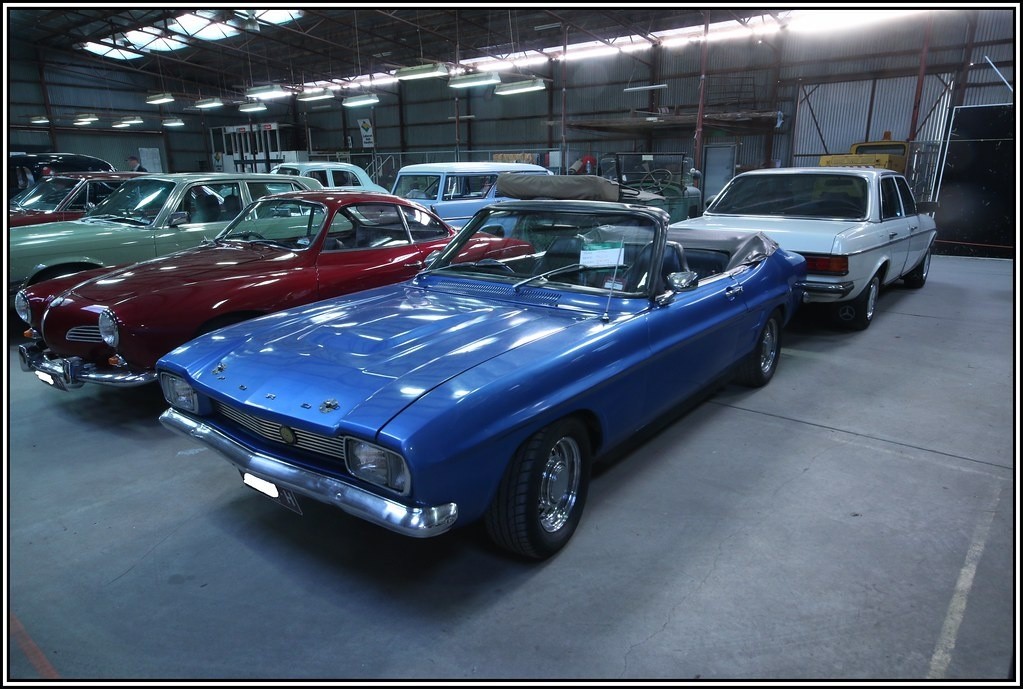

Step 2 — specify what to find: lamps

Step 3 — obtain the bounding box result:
[494,9,546,96]
[534,22,562,31]
[244,34,284,97]
[30,116,50,124]
[120,115,145,124]
[194,93,225,109]
[394,9,448,81]
[623,49,668,92]
[161,117,185,127]
[74,114,99,122]
[111,120,130,128]
[296,86,335,102]
[237,24,268,113]
[73,120,91,125]
[341,10,381,108]
[446,9,503,89]
[145,47,174,105]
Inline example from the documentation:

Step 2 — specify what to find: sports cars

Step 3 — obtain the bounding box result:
[153,176,809,566]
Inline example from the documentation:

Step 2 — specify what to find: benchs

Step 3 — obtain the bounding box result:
[355,225,443,247]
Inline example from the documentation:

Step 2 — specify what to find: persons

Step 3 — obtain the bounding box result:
[128,156,148,172]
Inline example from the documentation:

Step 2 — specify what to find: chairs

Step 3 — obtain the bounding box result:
[322,237,345,249]
[217,195,242,221]
[531,234,601,287]
[620,240,690,294]
[760,191,798,215]
[813,192,860,218]
[190,194,220,222]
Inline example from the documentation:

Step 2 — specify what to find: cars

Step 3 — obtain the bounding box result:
[14,195,542,395]
[659,165,942,333]
[8,152,705,330]
